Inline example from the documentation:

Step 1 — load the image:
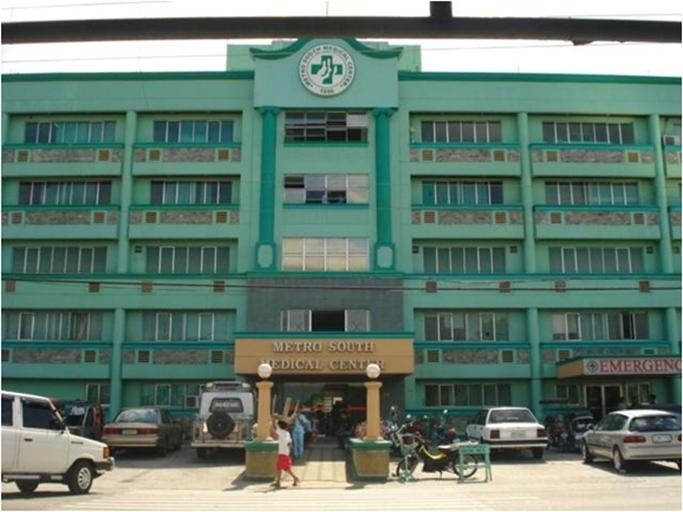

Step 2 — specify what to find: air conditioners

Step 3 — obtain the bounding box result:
[663,135,680,147]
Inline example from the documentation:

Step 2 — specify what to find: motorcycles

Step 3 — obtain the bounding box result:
[543,415,597,450]
[380,404,478,479]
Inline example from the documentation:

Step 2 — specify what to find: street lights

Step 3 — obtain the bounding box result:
[252,363,274,441]
[361,363,384,440]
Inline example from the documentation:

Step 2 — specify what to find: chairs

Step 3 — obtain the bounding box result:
[455,444,492,483]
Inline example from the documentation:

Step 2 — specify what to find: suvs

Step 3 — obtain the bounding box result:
[189,380,256,459]
[1,390,116,495]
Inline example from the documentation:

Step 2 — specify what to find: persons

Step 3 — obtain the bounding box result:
[648,394,656,407]
[268,413,298,486]
[618,396,626,409]
[69,398,85,415]
[630,395,640,408]
[315,404,324,418]
[290,404,311,455]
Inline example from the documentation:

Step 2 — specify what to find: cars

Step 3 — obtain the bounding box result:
[464,405,549,459]
[581,408,682,473]
[100,406,184,458]
